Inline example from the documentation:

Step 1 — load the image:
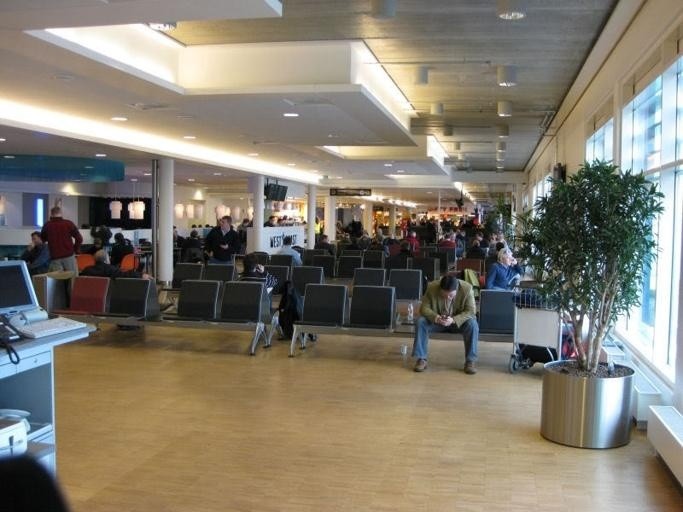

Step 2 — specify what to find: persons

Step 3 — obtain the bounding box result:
[139,238,151,256]
[238,253,277,315]
[82,223,90,229]
[275,236,302,266]
[79,237,121,278]
[314,235,336,256]
[336,213,509,271]
[411,275,478,376]
[484,247,524,291]
[173,224,214,267]
[204,216,241,265]
[21,231,50,278]
[40,207,83,295]
[237,214,320,246]
[109,233,134,265]
[90,223,112,253]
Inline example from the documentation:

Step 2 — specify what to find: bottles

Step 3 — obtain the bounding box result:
[608,360,614,376]
[407,301,413,320]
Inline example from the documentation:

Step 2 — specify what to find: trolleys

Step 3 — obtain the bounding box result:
[509,281,581,372]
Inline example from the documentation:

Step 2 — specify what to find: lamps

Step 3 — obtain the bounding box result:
[108,153,302,222]
[367,2,527,175]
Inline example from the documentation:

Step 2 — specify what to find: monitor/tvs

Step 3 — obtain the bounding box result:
[276,186,287,201]
[0,260,39,316]
[266,184,279,201]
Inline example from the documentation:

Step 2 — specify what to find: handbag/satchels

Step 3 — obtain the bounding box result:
[463,269,480,299]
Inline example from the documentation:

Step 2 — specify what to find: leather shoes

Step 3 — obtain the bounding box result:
[464,361,476,374]
[415,359,429,372]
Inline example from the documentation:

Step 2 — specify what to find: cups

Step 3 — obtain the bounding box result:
[399,344,408,355]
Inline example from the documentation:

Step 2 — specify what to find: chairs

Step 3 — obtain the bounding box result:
[0,222,515,357]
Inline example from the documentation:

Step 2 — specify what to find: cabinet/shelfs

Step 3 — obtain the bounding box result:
[0,316,96,482]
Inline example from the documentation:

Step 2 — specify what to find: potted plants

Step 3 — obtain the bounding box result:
[502,157,666,450]
